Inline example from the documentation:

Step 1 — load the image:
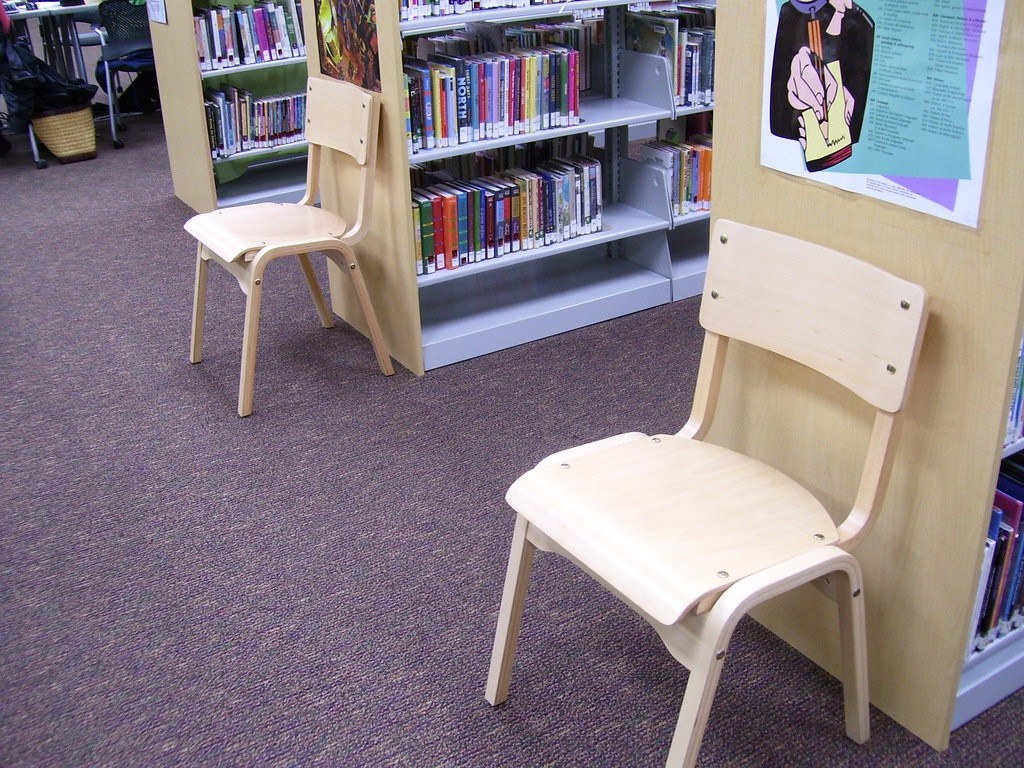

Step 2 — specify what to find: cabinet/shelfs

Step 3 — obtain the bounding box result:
[702,1,1024,751]
[301,0,720,376]
[146,0,308,214]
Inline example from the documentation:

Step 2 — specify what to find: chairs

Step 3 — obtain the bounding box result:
[183,77,394,417]
[35,0,161,149]
[486,220,930,768]
[0,0,48,169]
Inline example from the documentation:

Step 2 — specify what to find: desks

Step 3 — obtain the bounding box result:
[6,2,100,84]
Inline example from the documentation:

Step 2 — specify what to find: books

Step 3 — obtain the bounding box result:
[621,0,717,108]
[1002,336,1024,449]
[638,110,712,220]
[408,131,604,279]
[398,0,605,155]
[193,0,308,73]
[203,81,307,161]
[968,451,1024,656]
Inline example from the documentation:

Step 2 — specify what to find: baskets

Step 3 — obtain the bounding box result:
[29,98,97,164]
